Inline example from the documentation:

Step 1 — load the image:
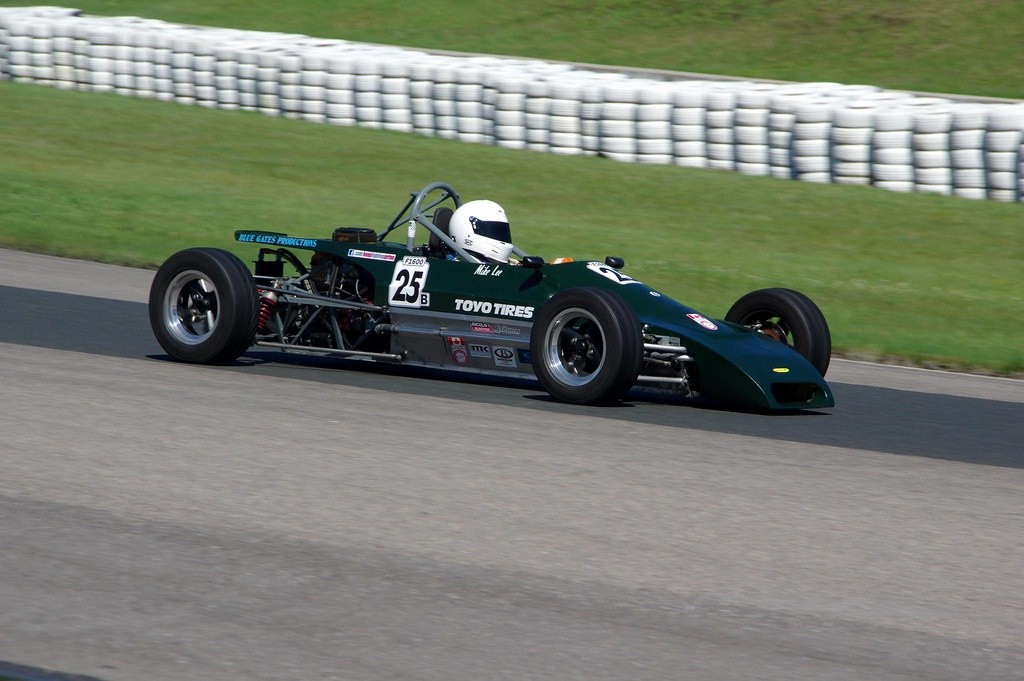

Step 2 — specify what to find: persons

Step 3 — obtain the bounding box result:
[448,196,526,265]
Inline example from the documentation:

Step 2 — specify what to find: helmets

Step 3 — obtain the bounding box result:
[450,199,513,264]
